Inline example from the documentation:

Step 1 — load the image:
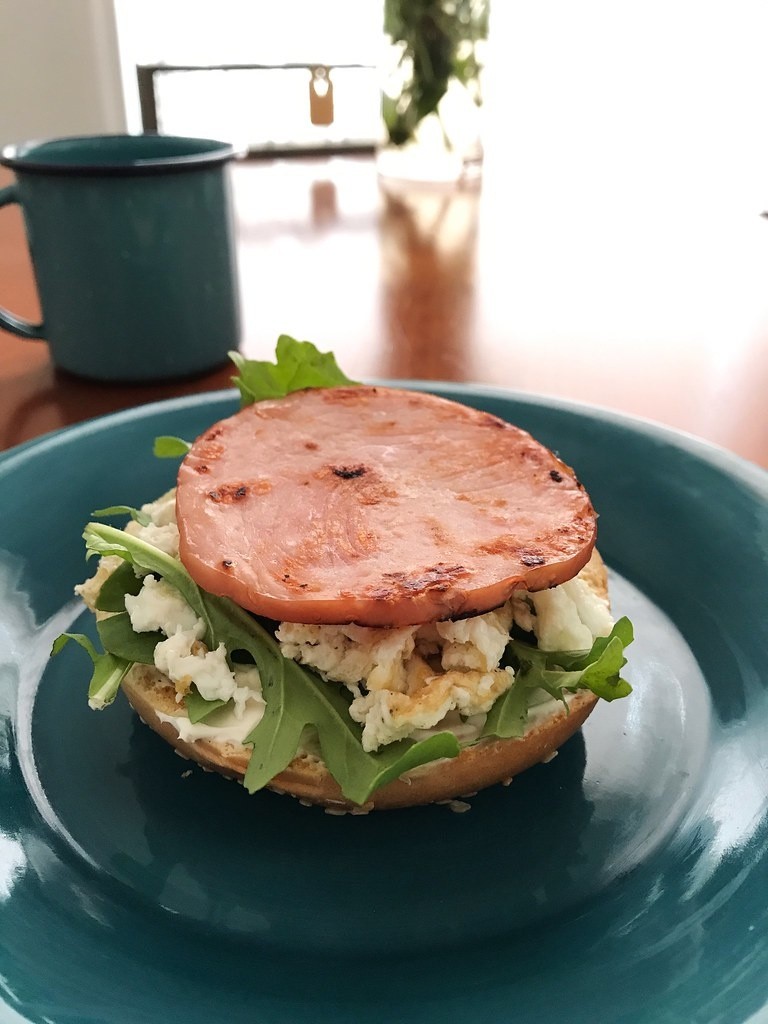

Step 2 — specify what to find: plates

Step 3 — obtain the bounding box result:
[0,379,768,1024]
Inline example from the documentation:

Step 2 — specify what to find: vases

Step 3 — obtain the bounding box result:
[374,0,493,179]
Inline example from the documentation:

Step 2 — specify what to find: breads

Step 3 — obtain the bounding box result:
[92,604,599,815]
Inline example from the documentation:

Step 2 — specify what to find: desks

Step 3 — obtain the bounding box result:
[0,157,768,474]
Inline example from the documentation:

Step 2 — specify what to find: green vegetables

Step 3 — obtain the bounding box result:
[49,333,635,805]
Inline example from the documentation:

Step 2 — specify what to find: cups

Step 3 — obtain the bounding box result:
[0,133,239,384]
